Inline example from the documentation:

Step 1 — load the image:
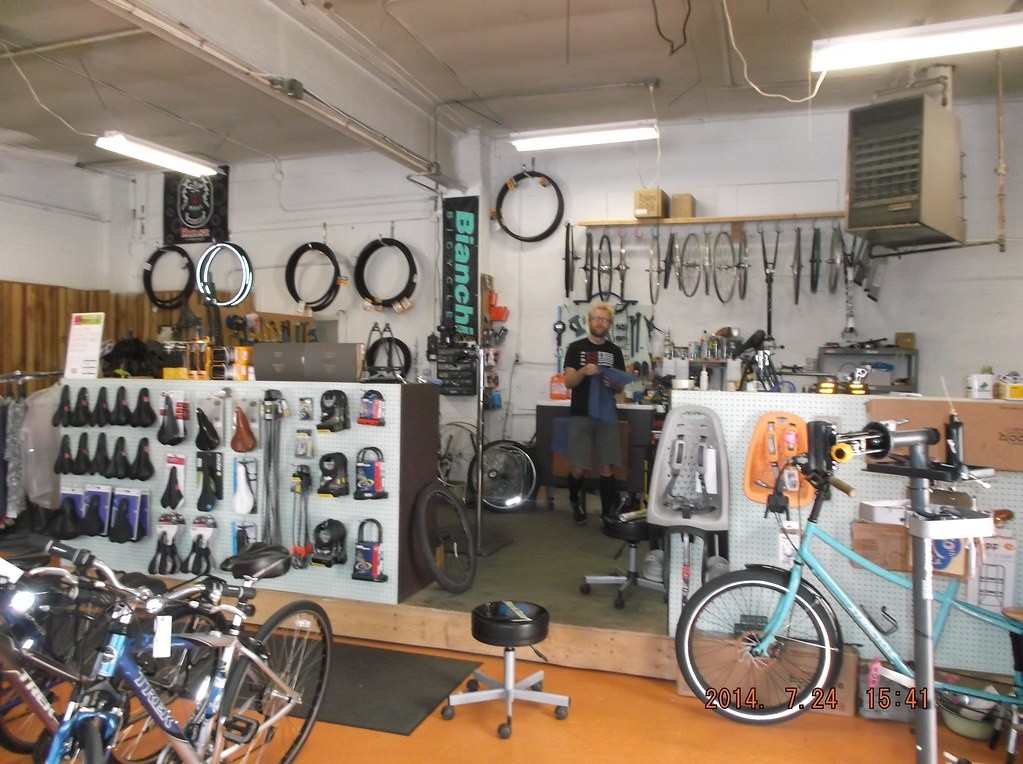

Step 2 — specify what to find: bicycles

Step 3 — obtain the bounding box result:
[674,463,1023,726]
[0,538,334,764]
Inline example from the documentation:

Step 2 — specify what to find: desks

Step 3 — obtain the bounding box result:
[535,399,656,511]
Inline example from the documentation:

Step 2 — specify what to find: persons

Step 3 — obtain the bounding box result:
[564,304,627,526]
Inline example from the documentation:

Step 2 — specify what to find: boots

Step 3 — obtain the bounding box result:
[567,474,588,525]
[598,474,617,525]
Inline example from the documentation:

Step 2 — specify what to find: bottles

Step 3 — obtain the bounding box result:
[708,333,719,359]
[699,365,709,391]
[700,330,709,359]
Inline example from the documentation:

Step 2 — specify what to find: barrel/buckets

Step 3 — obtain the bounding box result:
[550,373,570,400]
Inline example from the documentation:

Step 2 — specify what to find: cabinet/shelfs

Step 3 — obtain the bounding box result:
[818,343,921,399]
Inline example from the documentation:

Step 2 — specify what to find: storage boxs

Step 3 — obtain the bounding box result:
[994,380,1023,401]
[633,187,670,220]
[851,518,909,574]
[895,331,916,349]
[865,398,1023,477]
[966,374,993,399]
[910,530,970,579]
[861,671,920,723]
[671,194,695,218]
[677,628,859,723]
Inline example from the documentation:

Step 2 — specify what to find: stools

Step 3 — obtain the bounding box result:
[581,506,668,613]
[439,596,577,743]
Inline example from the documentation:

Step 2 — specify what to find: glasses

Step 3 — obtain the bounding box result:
[589,317,612,323]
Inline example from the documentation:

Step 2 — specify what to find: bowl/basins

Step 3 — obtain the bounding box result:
[937,697,997,741]
[671,379,695,389]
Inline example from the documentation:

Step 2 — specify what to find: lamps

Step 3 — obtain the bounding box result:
[508,77,661,154]
[808,9,1023,76]
[94,127,235,180]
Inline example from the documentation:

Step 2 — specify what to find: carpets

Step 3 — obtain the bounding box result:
[17,608,484,738]
